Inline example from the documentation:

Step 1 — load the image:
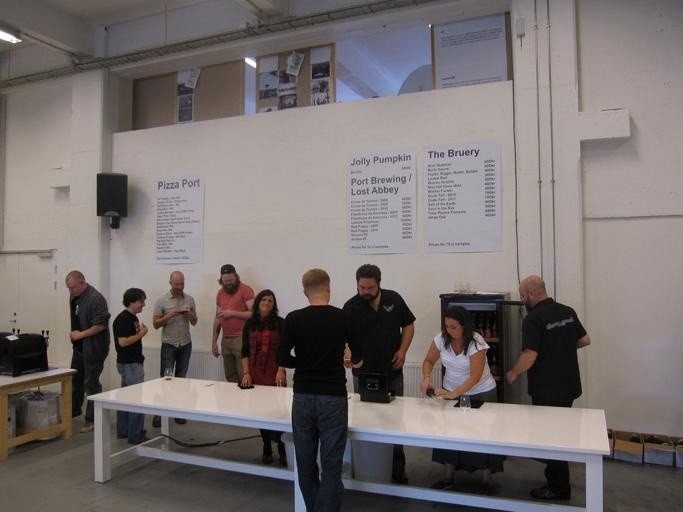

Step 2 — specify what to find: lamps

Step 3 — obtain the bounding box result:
[0,22,22,45]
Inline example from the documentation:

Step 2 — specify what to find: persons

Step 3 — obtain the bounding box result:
[66,270,111,433]
[419,305,508,494]
[153,272,199,428]
[507,275,591,504]
[113,288,150,445]
[343,264,416,484]
[240,289,287,466]
[212,264,256,382]
[274,269,363,510]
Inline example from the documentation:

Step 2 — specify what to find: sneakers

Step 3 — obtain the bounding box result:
[472,483,496,495]
[129,438,150,444]
[73,416,82,423]
[118,434,128,439]
[175,419,187,424]
[79,423,94,433]
[431,478,456,489]
[152,423,162,428]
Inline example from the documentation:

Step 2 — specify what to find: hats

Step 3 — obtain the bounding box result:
[221,264,236,275]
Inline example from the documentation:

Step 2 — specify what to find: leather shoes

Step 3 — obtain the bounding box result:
[530,487,572,501]
[263,447,274,465]
[391,472,408,485]
[278,446,287,468]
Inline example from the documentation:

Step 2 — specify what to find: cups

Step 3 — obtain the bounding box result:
[275,375,283,389]
[165,368,172,380]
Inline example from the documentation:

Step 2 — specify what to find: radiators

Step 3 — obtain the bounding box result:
[141,345,442,399]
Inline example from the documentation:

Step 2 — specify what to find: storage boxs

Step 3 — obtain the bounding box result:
[671,436,683,468]
[602,428,614,459]
[614,430,643,465]
[641,432,675,468]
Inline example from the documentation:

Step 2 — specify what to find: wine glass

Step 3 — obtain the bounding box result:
[459,396,471,421]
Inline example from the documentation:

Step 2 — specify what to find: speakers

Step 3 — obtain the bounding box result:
[97,172,128,219]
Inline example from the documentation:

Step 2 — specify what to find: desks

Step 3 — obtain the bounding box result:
[88,374,611,512]
[0,366,78,463]
